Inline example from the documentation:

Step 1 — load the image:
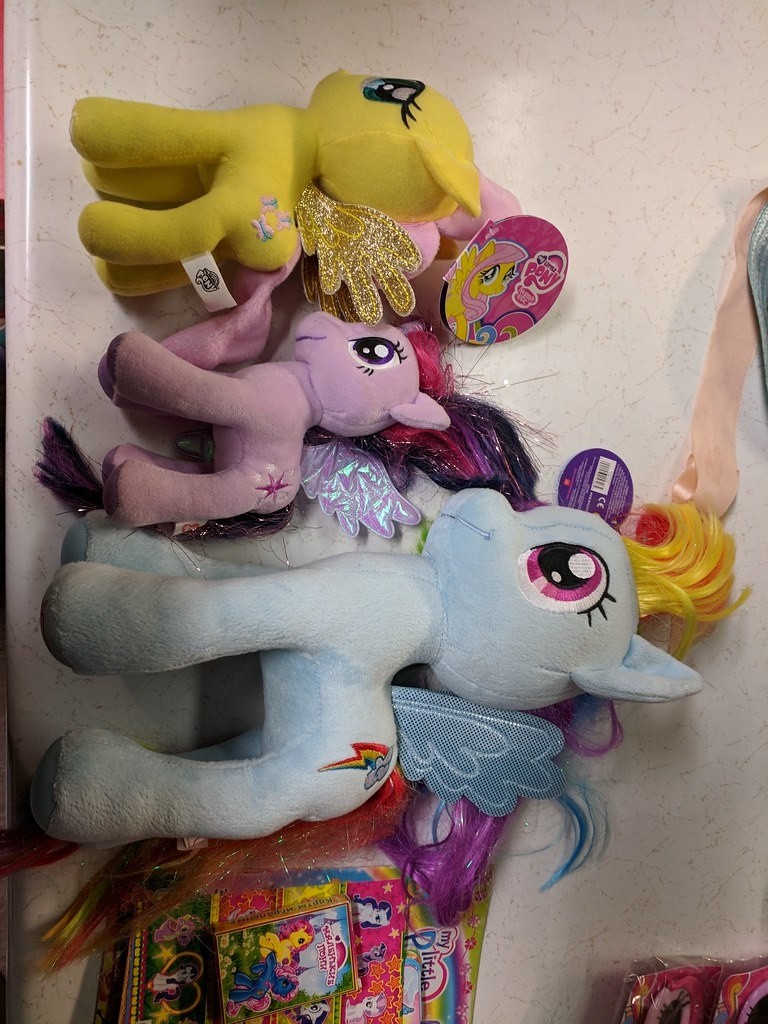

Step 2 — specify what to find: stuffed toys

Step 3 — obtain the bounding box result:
[30,68,754,843]
